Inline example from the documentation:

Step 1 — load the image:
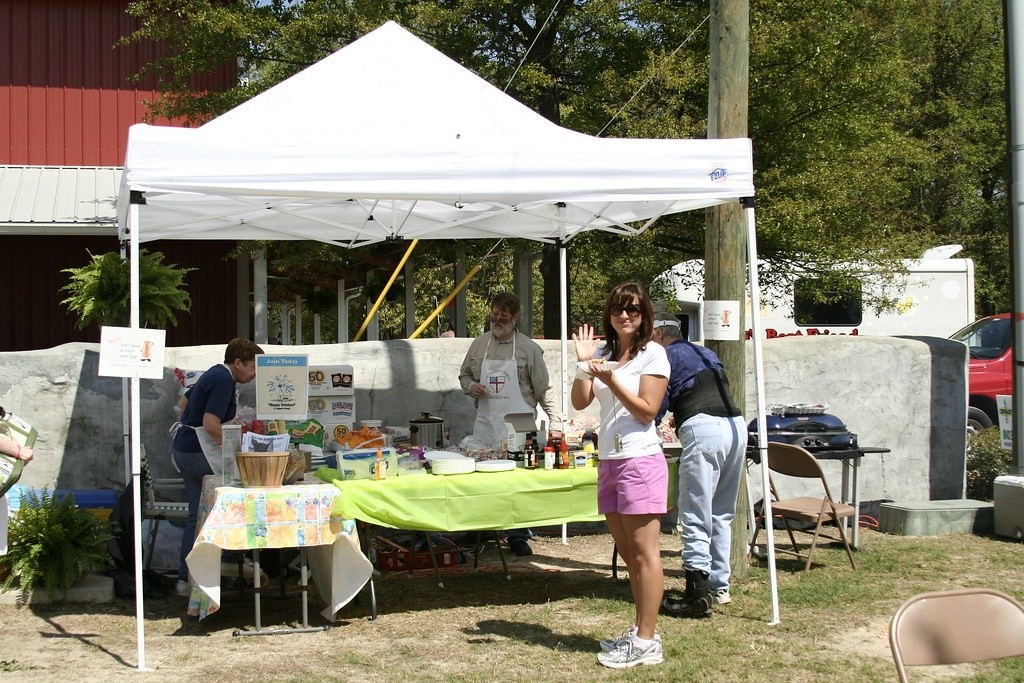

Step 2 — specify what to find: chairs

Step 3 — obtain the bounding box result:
[140,443,189,577]
[746,442,857,575]
[889,588,1024,683]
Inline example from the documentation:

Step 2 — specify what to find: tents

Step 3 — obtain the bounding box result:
[115,21,793,670]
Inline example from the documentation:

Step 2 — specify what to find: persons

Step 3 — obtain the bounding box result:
[651,311,748,614]
[458,292,562,451]
[572,279,673,667]
[172,335,268,597]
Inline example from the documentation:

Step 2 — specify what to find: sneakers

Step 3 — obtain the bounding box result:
[708,587,731,605]
[663,595,712,615]
[596,637,664,668]
[600,624,661,652]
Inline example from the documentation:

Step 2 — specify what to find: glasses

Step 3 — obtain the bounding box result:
[609,303,641,317]
[489,316,512,324]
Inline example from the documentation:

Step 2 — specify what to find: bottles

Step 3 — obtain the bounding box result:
[524,431,539,469]
[544,432,574,469]
[361,419,382,430]
[583,435,595,467]
[375,446,386,480]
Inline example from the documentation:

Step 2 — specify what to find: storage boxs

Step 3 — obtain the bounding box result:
[503,412,538,453]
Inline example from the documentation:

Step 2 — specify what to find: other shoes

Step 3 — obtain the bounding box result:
[453,532,490,546]
[510,539,533,557]
[176,580,191,595]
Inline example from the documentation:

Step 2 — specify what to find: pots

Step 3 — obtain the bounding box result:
[408,412,444,449]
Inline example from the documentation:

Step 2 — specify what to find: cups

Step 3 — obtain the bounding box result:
[224,439,240,482]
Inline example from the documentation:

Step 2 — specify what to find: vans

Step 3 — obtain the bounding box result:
[649,241,979,360]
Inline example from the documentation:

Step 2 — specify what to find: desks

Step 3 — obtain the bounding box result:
[183,474,374,637]
[316,449,680,616]
[743,446,890,550]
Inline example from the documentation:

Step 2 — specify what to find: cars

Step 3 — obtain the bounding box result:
[946,313,1015,448]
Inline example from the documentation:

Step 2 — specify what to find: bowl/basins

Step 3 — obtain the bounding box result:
[423,451,464,466]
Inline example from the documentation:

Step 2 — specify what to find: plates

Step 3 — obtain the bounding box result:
[310,452,335,469]
[577,360,620,372]
[431,457,516,471]
